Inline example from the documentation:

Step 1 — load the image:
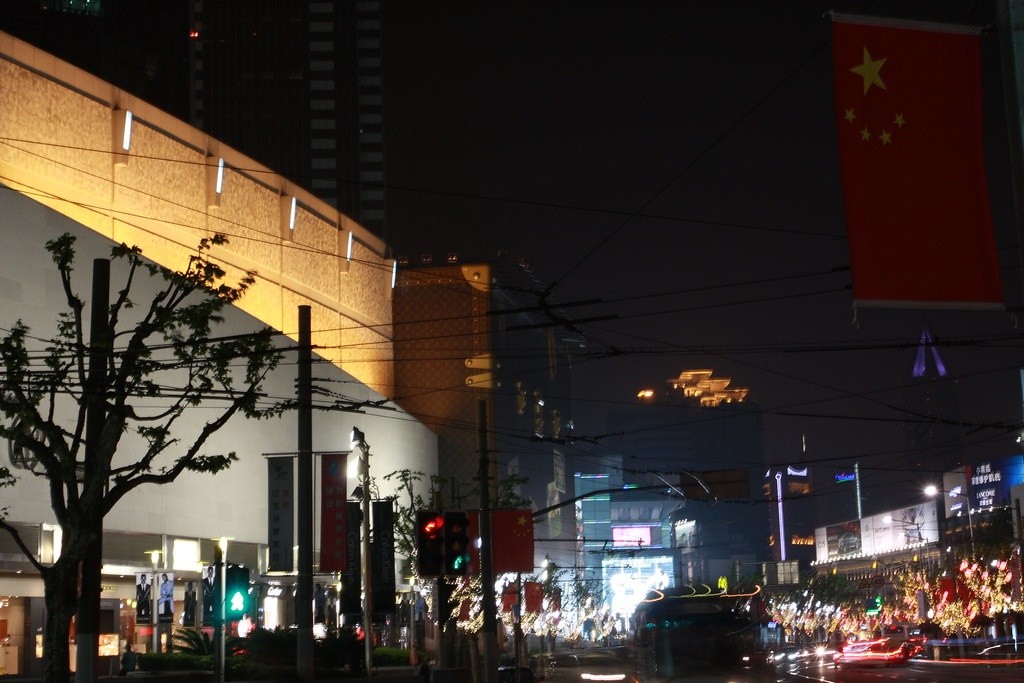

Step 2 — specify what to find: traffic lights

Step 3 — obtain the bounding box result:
[876,596,883,608]
[443,511,472,577]
[416,510,444,577]
[225,565,251,622]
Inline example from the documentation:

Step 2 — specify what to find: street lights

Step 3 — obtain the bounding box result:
[882,515,925,591]
[144,549,167,654]
[348,425,373,683]
[924,486,985,648]
[190,560,211,634]
[210,536,237,683]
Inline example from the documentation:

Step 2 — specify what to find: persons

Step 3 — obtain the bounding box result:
[203,565,216,596]
[326,598,337,630]
[312,583,327,623]
[184,581,197,627]
[118,643,138,676]
[261,614,411,654]
[160,574,174,616]
[136,573,151,618]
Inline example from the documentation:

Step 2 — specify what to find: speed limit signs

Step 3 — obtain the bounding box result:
[871,575,885,589]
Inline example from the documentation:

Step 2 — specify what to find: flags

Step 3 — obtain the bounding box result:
[503,582,516,613]
[490,511,534,574]
[524,582,544,613]
